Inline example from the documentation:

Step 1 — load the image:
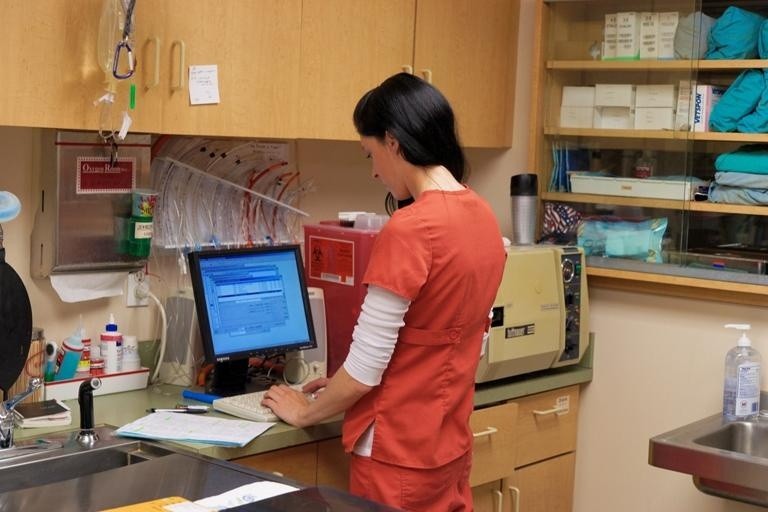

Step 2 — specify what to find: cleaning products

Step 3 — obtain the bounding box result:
[722,322,762,419]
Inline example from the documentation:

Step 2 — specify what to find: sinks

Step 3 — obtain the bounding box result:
[648,410,768,507]
[0,441,300,512]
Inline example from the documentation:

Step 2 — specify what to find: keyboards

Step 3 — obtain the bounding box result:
[213,388,316,422]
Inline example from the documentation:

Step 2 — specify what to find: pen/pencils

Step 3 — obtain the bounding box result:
[151,405,209,413]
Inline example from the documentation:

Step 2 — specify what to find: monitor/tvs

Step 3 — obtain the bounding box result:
[187,245,317,395]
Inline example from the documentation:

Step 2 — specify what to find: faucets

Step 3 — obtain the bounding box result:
[0,376,41,449]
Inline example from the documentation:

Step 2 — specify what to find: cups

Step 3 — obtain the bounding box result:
[131,184,158,219]
[509,172,540,246]
[126,217,157,258]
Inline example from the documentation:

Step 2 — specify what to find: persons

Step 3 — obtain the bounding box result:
[263,73,506,512]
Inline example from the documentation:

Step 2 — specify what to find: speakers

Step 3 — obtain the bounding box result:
[286,287,328,387]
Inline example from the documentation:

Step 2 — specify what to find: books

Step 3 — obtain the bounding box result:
[113,410,275,448]
[12,398,72,429]
[103,480,329,512]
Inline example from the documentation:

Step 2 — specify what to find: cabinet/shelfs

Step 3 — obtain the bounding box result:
[1,0,520,151]
[524,2,767,305]
[439,382,584,512]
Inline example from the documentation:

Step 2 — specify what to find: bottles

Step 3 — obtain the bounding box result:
[47,313,142,383]
